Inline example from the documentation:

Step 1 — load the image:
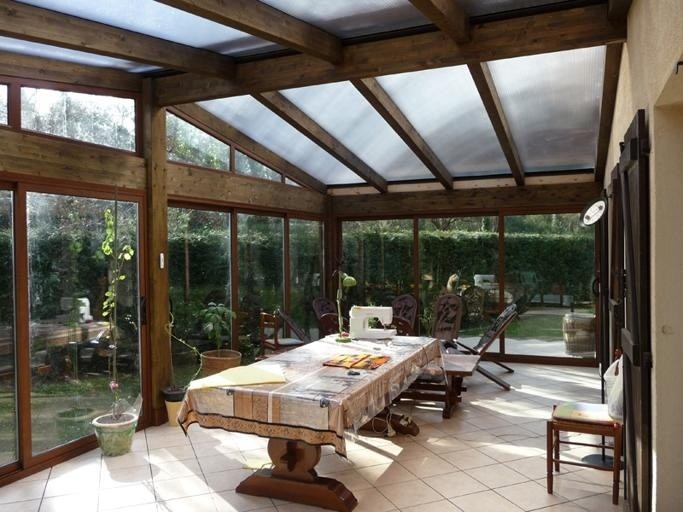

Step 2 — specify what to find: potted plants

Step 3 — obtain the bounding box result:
[192,302,242,378]
[91,206,138,457]
[160,312,204,401]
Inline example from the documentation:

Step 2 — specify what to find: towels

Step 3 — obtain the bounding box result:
[190,362,286,390]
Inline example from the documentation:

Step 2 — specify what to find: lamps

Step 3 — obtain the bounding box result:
[579,189,608,228]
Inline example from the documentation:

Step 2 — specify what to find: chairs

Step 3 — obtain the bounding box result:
[547,349,624,505]
[258,286,518,391]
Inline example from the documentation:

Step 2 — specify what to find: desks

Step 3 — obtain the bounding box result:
[177,332,449,512]
[392,353,481,419]
